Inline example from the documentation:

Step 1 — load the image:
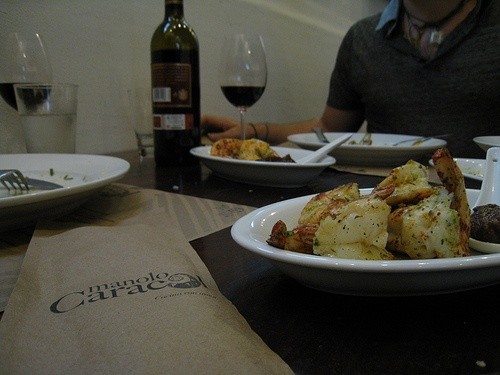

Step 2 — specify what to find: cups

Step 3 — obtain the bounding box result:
[127,88,154,159]
[13,84,78,153]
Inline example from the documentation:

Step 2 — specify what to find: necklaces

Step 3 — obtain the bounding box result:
[403,0,470,49]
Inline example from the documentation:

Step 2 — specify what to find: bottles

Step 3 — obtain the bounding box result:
[150,1,201,188]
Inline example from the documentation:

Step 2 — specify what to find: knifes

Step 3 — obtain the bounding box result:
[15,176,64,188]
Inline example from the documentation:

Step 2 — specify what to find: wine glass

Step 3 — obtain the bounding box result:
[217,33,269,140]
[0,31,52,154]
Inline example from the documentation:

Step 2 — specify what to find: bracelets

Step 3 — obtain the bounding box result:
[248,121,258,139]
[264,123,269,141]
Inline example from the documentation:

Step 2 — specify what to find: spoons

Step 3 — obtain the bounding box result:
[467,147,500,254]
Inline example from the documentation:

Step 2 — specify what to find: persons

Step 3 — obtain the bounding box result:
[201,0,500,188]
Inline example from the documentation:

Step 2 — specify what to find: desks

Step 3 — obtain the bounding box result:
[0,146,499,375]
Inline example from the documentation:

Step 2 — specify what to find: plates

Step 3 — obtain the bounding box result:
[287,132,448,166]
[0,154,131,231]
[428,158,485,180]
[189,146,337,188]
[228,187,500,297]
[473,136,500,153]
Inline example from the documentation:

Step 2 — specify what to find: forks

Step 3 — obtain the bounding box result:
[0,169,30,191]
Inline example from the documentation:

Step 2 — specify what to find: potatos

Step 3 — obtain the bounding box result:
[213,138,280,161]
[296,156,464,259]
